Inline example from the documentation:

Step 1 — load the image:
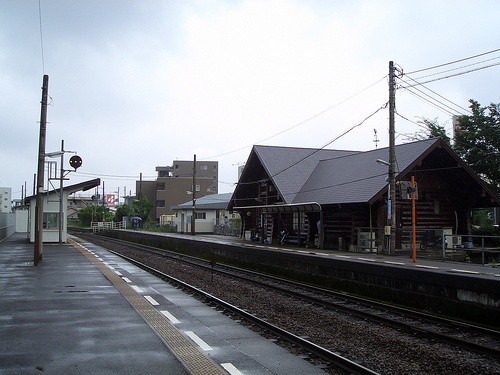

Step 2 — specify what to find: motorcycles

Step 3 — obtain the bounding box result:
[418,232,447,251]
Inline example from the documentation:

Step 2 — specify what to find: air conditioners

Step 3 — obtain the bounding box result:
[359,232,375,249]
[451,236,461,246]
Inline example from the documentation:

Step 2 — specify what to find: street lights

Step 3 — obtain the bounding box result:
[375,159,392,252]
